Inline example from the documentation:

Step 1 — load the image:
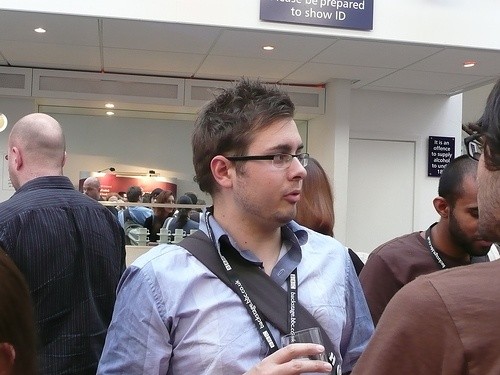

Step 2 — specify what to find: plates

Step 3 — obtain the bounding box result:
[172,234,186,236]
[135,240,150,242]
[157,234,172,236]
[157,240,170,243]
[135,233,150,235]
[171,241,181,244]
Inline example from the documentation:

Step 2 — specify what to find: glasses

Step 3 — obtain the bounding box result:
[464,132,486,161]
[141,195,144,198]
[226,153,309,169]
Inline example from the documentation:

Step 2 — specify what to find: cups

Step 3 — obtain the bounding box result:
[281,327,331,375]
[175,229,186,234]
[160,236,171,242]
[174,236,183,242]
[138,242,146,247]
[139,228,149,233]
[160,229,171,234]
[190,230,198,234]
[138,235,149,240]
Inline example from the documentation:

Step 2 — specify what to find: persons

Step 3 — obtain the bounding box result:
[293,157,364,275]
[0,113,127,375]
[358,152,500,329]
[350,77,500,375]
[83,176,207,245]
[0,246,45,375]
[96,77,376,375]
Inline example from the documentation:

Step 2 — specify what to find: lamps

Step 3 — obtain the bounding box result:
[98,167,115,172]
